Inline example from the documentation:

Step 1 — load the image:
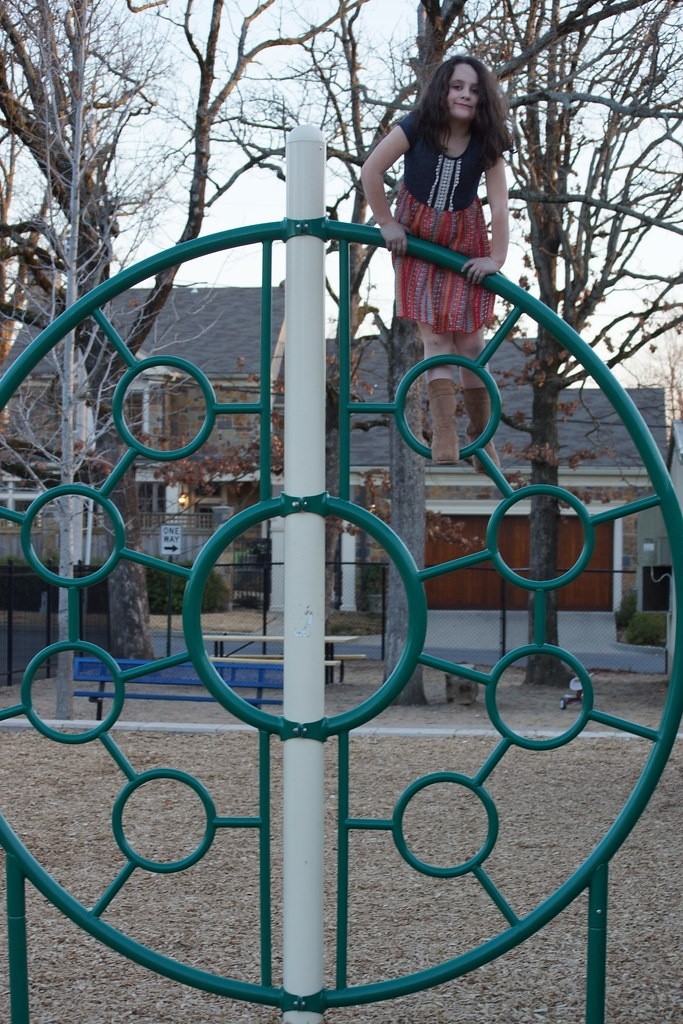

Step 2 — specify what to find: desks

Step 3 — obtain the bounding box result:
[203,635,359,683]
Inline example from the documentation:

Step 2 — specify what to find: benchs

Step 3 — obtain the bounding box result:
[208,654,367,684]
[74,658,284,721]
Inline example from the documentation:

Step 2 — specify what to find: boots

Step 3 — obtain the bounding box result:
[464,388,500,473]
[428,378,459,464]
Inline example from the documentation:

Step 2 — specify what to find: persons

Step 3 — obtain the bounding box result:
[362,56,509,471]
